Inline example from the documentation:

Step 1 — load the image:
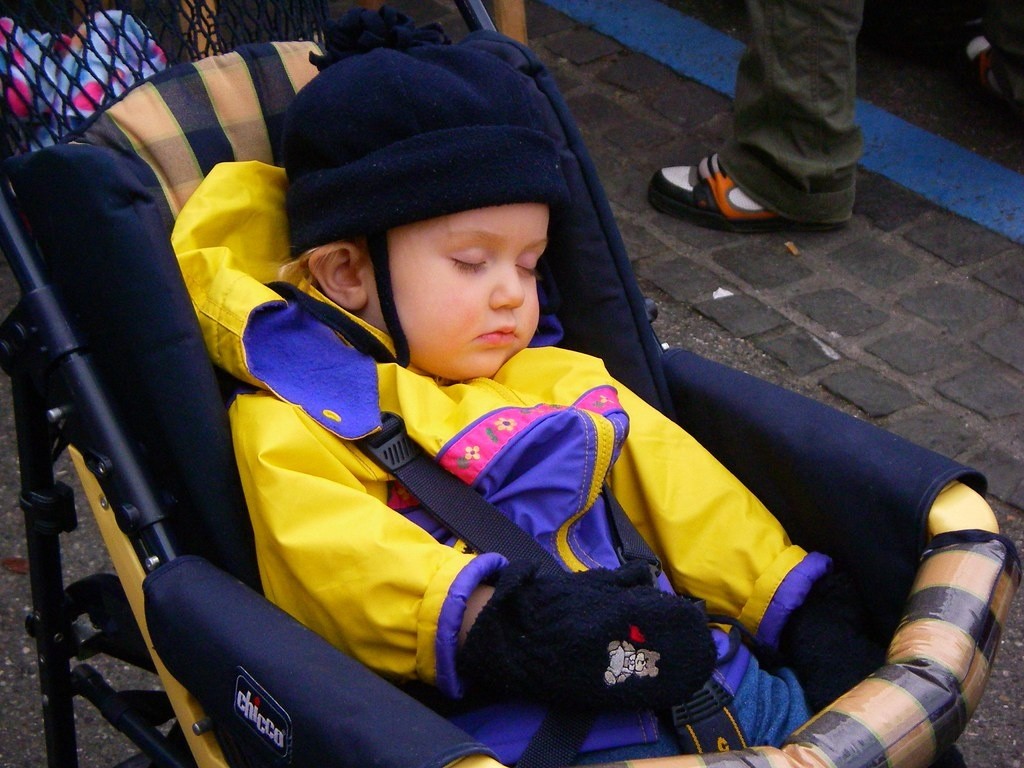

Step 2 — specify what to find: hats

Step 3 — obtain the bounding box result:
[276,2,576,374]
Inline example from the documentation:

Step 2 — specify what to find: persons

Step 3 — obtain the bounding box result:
[648,0,1024,233]
[170,5,833,768]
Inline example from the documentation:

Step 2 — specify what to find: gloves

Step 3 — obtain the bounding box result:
[453,558,723,718]
[785,599,889,712]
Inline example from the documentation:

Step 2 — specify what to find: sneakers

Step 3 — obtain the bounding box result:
[646,152,848,233]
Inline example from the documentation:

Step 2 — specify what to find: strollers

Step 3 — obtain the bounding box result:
[2,1,1022,768]
